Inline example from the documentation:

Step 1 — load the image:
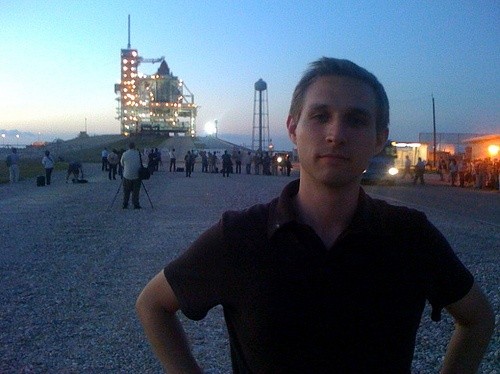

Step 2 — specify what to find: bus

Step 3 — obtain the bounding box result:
[360,140,398,186]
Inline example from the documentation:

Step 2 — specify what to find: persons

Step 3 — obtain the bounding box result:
[100,143,293,181]
[42,150,56,186]
[6,147,20,183]
[121,142,149,210]
[65,160,84,184]
[135,57,496,374]
[380,147,500,192]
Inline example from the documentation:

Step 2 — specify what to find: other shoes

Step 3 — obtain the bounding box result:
[123,204,127,209]
[134,205,141,209]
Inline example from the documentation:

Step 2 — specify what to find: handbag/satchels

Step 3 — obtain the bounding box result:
[138,168,150,180]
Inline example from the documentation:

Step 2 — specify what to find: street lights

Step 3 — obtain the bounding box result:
[2,134,5,147]
[16,134,19,148]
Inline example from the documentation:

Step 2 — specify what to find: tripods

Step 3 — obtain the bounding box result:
[109,166,156,210]
